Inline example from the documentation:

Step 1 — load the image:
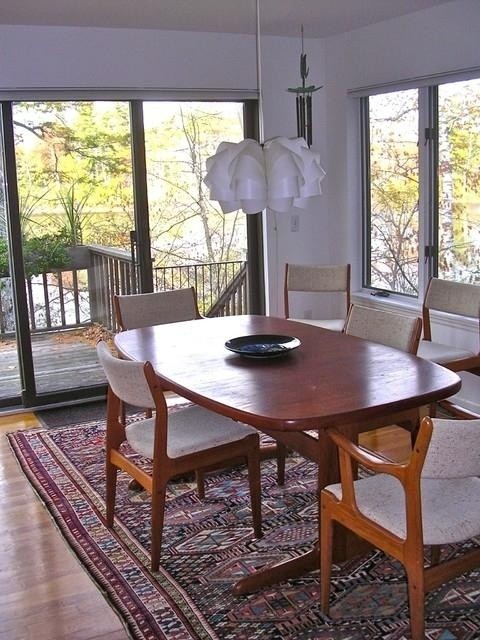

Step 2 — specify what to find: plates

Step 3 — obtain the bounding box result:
[225,334,302,359]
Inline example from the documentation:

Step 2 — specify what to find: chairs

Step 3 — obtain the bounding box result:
[415,276,480,372]
[428,370,480,420]
[283,263,351,334]
[343,302,422,355]
[94,339,262,573]
[113,286,209,418]
[319,415,480,640]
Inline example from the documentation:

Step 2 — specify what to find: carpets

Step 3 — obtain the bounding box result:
[7,399,480,640]
[33,401,147,431]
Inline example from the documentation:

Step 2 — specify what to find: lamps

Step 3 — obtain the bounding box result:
[202,0,327,216]
